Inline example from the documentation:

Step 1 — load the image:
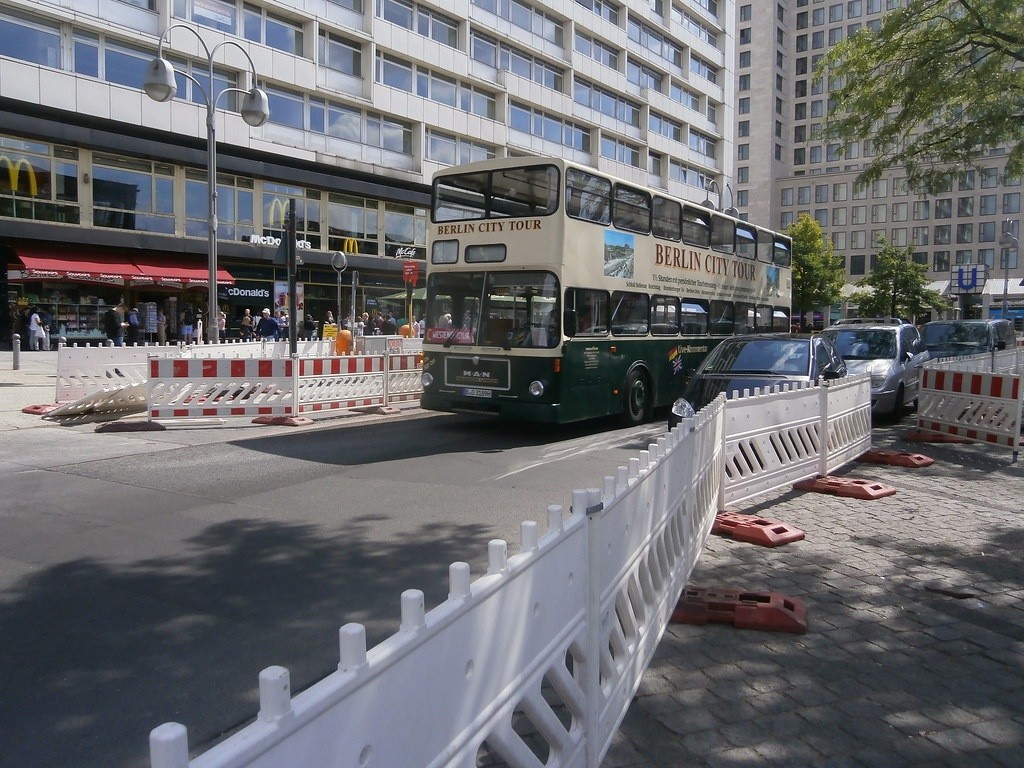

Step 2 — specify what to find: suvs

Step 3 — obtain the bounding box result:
[818,317,930,422]
[919,317,1017,361]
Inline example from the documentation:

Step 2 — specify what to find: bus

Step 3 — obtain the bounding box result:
[418,154,795,429]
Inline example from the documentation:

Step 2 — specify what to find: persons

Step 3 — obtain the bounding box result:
[344,314,351,330]
[240,315,256,342]
[273,311,290,342]
[439,310,452,328]
[157,307,166,346]
[324,310,333,324]
[245,309,253,326]
[218,311,226,338]
[19,306,52,350]
[106,303,129,346]
[413,316,425,337]
[356,310,396,336]
[791,321,800,333]
[806,323,813,334]
[127,308,140,346]
[256,308,278,341]
[301,314,316,341]
[179,307,193,344]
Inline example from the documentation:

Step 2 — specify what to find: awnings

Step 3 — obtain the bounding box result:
[14,246,236,285]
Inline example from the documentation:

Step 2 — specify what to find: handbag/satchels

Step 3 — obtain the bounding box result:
[34,324,47,339]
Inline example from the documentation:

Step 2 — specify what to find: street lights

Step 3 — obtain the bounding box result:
[144,23,271,343]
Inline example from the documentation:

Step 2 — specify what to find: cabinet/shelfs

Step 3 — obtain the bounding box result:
[30,301,119,335]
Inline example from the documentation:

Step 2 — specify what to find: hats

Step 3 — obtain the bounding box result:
[132,308,140,314]
[261,307,270,315]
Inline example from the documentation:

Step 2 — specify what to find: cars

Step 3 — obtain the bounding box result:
[666,333,848,432]
